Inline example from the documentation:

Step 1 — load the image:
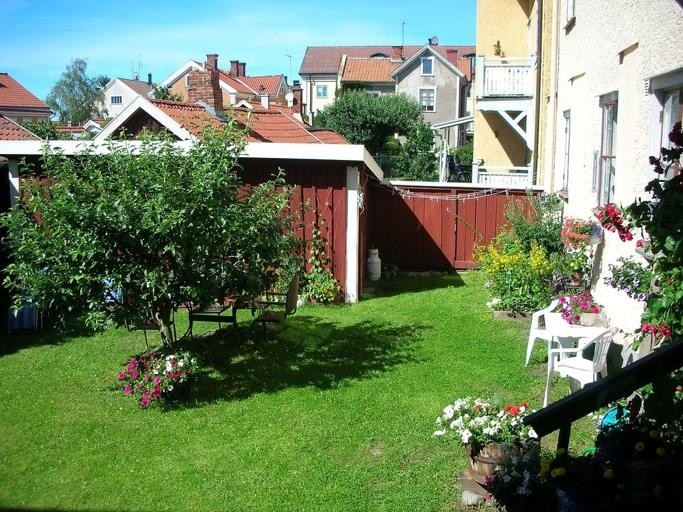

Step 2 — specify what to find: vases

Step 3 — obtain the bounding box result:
[590,224,604,245]
[161,380,190,402]
[464,442,503,485]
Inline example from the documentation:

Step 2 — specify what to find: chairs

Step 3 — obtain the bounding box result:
[525,295,619,417]
[7,277,54,335]
[120,281,178,354]
[249,274,303,349]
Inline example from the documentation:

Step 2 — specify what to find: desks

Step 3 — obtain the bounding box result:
[178,297,244,343]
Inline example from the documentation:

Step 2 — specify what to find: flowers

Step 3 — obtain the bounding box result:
[559,291,605,325]
[537,448,593,490]
[117,346,201,410]
[429,396,542,498]
[594,419,667,479]
[558,215,601,260]
